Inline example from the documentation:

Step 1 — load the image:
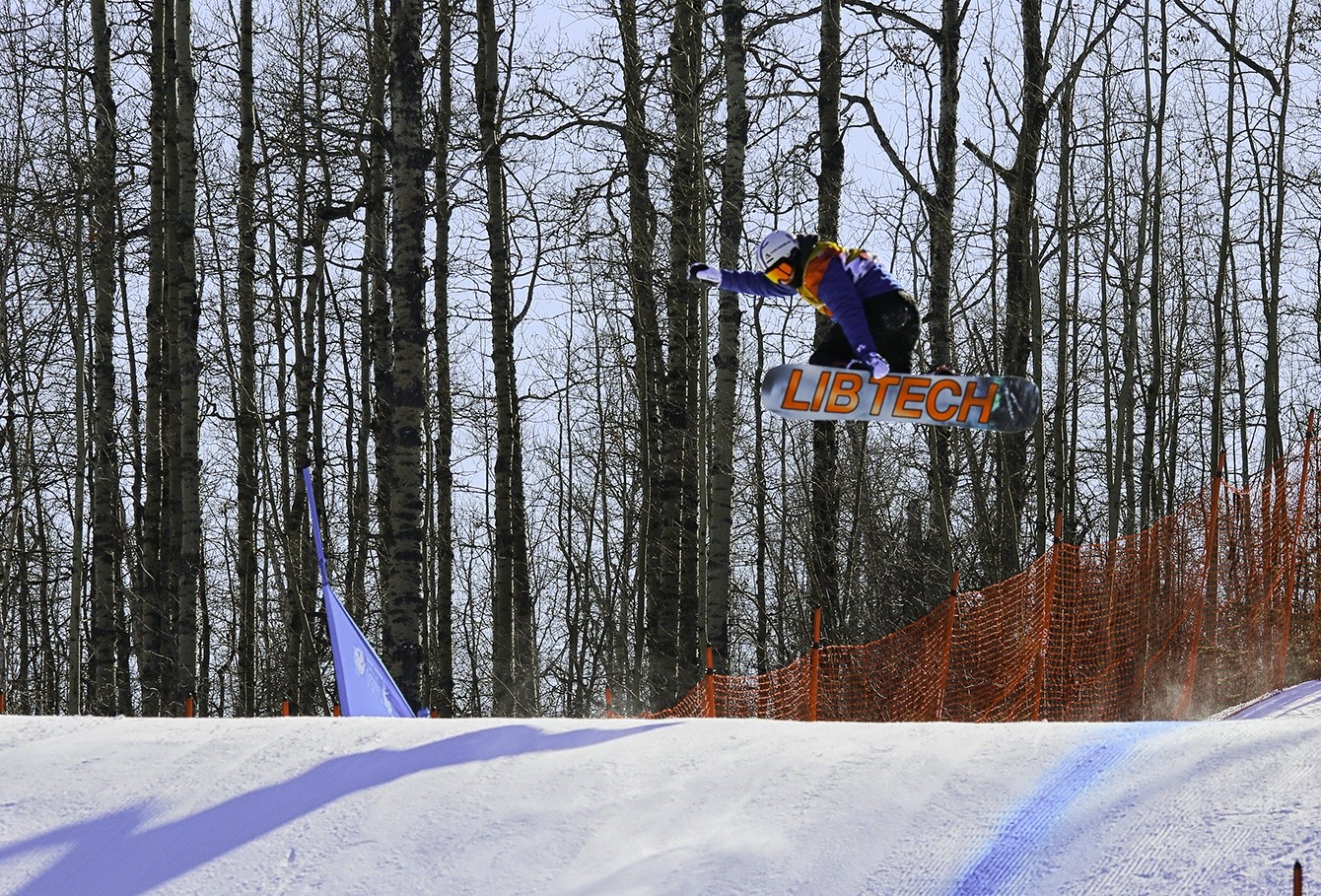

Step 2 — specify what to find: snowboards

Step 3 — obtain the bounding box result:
[758,362,1043,434]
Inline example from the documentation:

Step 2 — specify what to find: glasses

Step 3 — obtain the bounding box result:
[764,256,795,285]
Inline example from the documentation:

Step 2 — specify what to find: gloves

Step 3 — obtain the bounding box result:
[864,352,889,380]
[687,263,722,289]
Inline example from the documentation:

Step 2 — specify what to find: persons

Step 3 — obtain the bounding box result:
[686,230,955,380]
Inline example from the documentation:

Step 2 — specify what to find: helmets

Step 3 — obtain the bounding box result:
[756,230,801,286]
